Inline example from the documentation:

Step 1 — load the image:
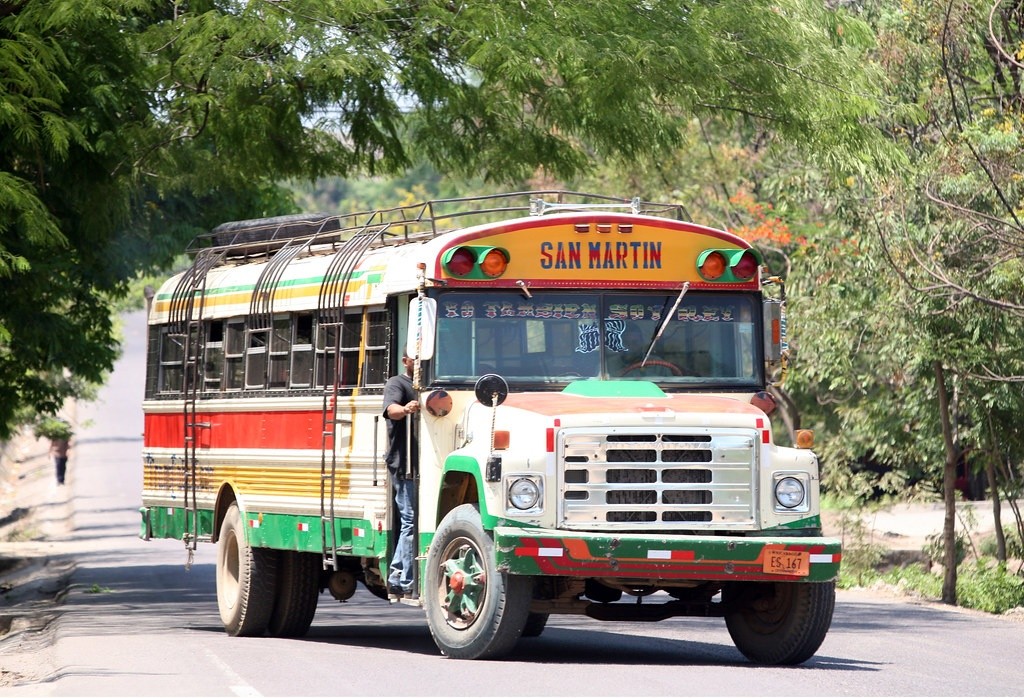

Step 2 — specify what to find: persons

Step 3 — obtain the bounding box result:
[49,439,75,486]
[381,342,423,601]
[596,321,671,379]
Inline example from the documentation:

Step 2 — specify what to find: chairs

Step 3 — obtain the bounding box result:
[438,330,497,375]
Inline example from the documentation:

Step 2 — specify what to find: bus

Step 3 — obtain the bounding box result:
[138,187,845,668]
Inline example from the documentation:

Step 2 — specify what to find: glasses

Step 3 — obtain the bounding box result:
[622,338,643,347]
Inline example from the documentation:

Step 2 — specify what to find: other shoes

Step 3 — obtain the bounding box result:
[404,590,413,599]
[387,583,404,599]
